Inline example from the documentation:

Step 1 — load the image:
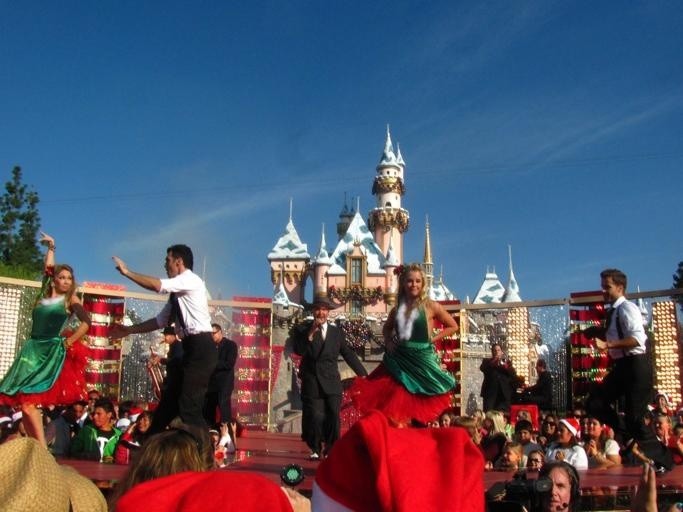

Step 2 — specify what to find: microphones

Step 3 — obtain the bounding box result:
[562,503,576,508]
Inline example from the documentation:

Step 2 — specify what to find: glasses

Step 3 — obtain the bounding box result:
[212,330,220,335]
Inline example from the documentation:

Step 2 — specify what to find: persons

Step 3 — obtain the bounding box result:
[147,325,184,438]
[290,296,368,463]
[104,427,209,512]
[581,268,674,477]
[1,230,92,454]
[202,323,238,429]
[478,343,515,412]
[107,242,219,437]
[0,384,237,468]
[518,358,553,412]
[277,484,311,512]
[379,264,459,397]
[411,387,681,512]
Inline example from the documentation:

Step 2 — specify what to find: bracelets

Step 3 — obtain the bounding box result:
[46,244,56,251]
[64,337,74,348]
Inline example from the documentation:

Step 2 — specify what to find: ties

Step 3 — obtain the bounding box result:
[167,292,186,330]
[603,308,614,336]
[313,323,325,361]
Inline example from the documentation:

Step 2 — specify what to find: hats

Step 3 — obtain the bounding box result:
[304,297,337,309]
[309,408,488,512]
[159,326,177,335]
[0,406,24,424]
[655,393,669,403]
[0,436,108,512]
[559,416,581,437]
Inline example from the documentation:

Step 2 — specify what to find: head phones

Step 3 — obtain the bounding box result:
[566,463,579,505]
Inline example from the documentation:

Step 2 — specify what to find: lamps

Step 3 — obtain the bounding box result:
[279,462,305,491]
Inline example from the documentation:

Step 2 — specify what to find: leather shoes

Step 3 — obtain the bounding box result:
[120,436,143,450]
[617,441,637,457]
[655,465,671,477]
[311,450,332,461]
